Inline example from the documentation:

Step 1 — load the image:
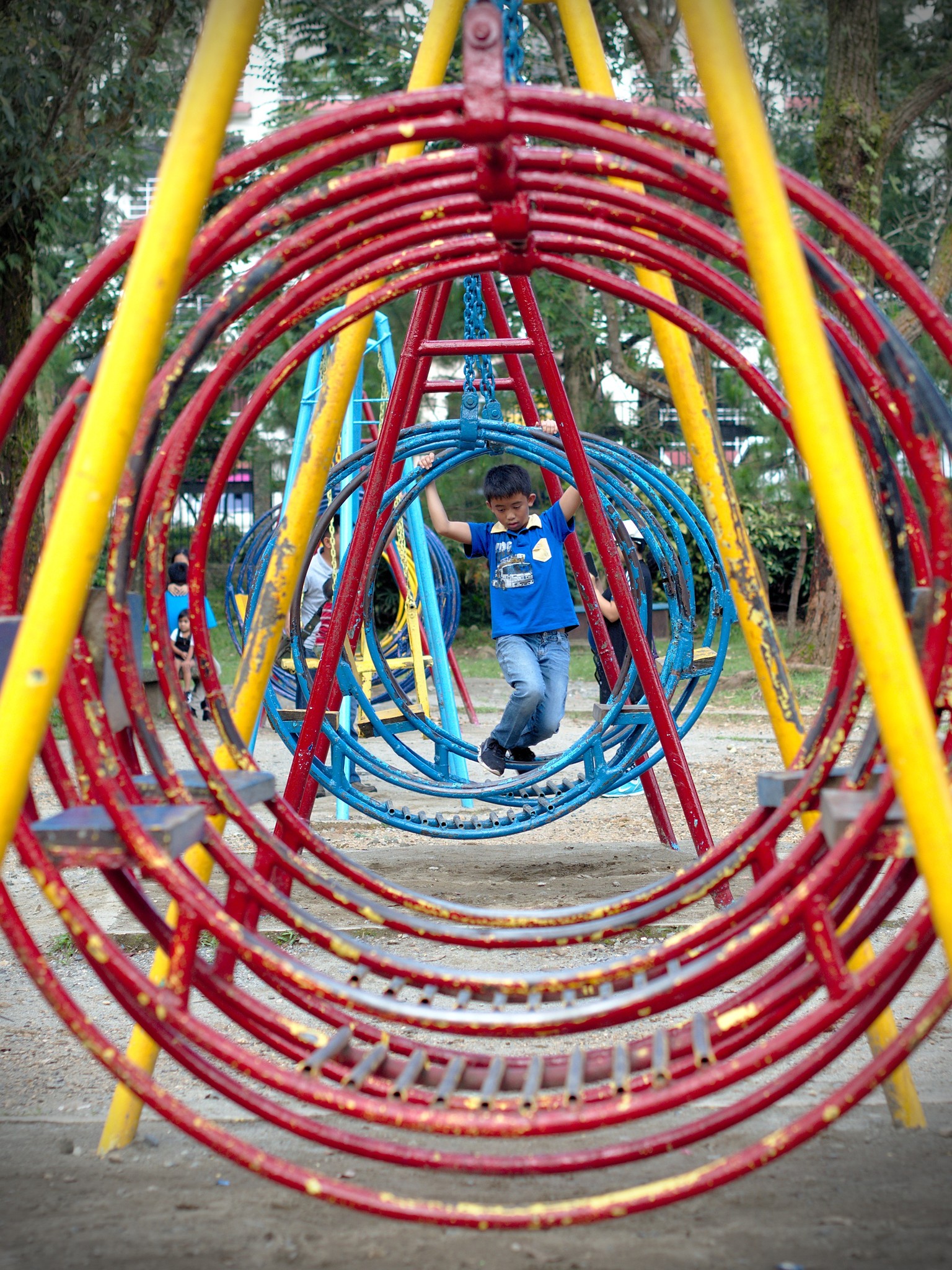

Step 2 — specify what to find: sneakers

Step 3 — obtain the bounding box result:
[601,776,644,798]
[509,745,537,776]
[478,736,507,776]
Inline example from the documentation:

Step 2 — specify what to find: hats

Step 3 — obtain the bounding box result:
[611,519,644,541]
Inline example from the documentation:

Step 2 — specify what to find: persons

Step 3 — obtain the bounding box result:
[170,609,196,705]
[288,514,377,795]
[314,578,359,738]
[143,548,221,722]
[585,520,656,797]
[410,417,584,776]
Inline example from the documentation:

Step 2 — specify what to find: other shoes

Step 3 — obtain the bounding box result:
[350,781,377,793]
[316,783,325,798]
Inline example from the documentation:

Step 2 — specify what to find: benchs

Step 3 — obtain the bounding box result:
[141,667,200,717]
